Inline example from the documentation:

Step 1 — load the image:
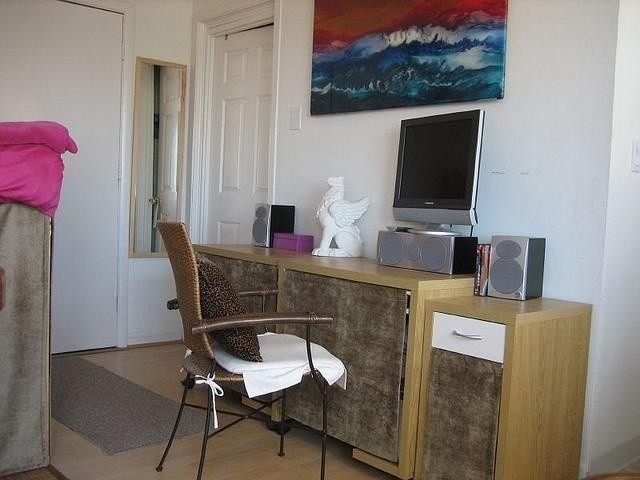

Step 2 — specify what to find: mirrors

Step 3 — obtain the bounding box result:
[128,55,187,259]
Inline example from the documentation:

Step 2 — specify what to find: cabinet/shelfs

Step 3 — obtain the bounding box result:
[414,295,593,480]
[192,243,477,479]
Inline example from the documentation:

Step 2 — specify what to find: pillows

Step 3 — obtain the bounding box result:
[193,249,264,364]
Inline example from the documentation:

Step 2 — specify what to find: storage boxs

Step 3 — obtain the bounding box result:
[273,232,314,252]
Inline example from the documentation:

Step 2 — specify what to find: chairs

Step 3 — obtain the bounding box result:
[154,219,348,480]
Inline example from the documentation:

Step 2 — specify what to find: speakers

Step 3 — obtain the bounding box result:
[253,204,296,247]
[487,234,545,300]
[376,231,477,275]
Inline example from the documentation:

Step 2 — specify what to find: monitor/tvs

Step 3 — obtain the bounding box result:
[387,109,485,237]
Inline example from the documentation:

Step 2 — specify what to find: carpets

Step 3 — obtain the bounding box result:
[50,354,222,455]
